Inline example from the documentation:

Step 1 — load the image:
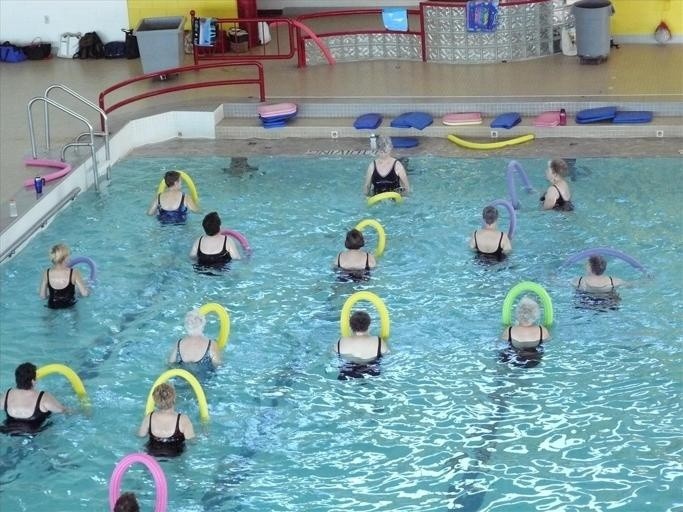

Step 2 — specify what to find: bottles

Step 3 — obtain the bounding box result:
[368,134,376,150]
[559,109,566,127]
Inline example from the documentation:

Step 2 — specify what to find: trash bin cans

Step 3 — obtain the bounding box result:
[573,0,615,65]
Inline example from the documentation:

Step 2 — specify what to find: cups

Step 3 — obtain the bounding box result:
[34,175,45,192]
[8,197,18,217]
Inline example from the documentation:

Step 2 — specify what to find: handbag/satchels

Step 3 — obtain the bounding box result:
[104,42,124,58]
[184,29,249,55]
[22,44,51,60]
[125,32,140,59]
[79,32,102,59]
[56,33,81,59]
[0,42,28,62]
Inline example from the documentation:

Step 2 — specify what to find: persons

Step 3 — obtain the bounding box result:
[186,211,252,273]
[137,383,198,462]
[552,254,646,313]
[365,135,413,201]
[146,170,201,226]
[334,228,378,285]
[496,294,552,370]
[332,309,389,380]
[38,242,92,308]
[0,362,90,436]
[114,490,141,512]
[512,157,578,219]
[166,310,223,380]
[468,204,512,264]
